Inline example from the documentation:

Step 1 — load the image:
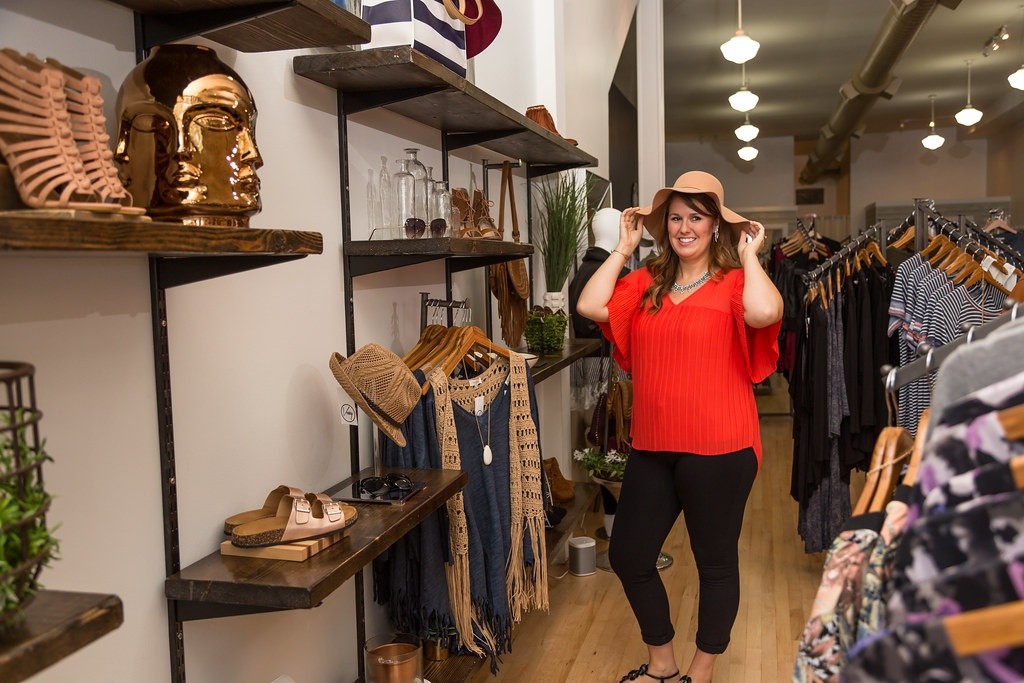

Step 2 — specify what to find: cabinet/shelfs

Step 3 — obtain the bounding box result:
[292,46,599,278]
[512,338,602,568]
[165,464,490,683]
[0,0,372,683]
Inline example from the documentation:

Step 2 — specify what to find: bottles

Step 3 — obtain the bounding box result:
[390,147,461,239]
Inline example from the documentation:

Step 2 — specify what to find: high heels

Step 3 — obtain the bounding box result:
[44,57,148,214]
[473,189,502,239]
[451,187,483,239]
[0,46,123,213]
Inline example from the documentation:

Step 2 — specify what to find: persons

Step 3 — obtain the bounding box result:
[575,171,784,683]
[563,207,636,539]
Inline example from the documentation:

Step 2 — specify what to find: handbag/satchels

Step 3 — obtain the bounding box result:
[488,255,529,349]
[586,379,633,453]
[361,0,484,79]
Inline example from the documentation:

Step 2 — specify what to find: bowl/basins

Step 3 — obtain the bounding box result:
[468,351,539,369]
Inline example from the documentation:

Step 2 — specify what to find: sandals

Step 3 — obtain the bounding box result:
[618,663,692,683]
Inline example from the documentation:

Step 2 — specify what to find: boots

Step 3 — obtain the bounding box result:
[527,105,578,146]
[543,457,576,499]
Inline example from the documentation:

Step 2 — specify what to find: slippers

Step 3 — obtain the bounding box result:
[224,485,333,535]
[231,494,357,546]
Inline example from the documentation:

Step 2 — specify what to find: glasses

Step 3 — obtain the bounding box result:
[360,472,412,498]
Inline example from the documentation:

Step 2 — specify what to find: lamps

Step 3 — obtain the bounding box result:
[728,63,759,112]
[720,0,761,65]
[737,141,759,162]
[921,94,945,151]
[955,58,984,126]
[1007,64,1024,90]
[983,25,1010,57]
[734,111,760,143]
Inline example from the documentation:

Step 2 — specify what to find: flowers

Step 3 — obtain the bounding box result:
[572,447,629,482]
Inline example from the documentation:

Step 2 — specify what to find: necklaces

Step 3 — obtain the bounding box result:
[671,272,711,293]
[458,338,496,465]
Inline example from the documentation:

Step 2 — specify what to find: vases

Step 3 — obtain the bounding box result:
[592,476,622,502]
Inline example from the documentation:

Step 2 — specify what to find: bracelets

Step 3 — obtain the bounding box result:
[609,249,629,263]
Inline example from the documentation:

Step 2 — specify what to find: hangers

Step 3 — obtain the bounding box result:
[400,296,510,396]
[779,198,1024,658]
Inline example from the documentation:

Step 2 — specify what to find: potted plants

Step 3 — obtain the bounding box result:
[417,621,458,662]
[519,168,599,314]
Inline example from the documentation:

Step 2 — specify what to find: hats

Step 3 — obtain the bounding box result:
[635,170,750,248]
[329,343,422,448]
